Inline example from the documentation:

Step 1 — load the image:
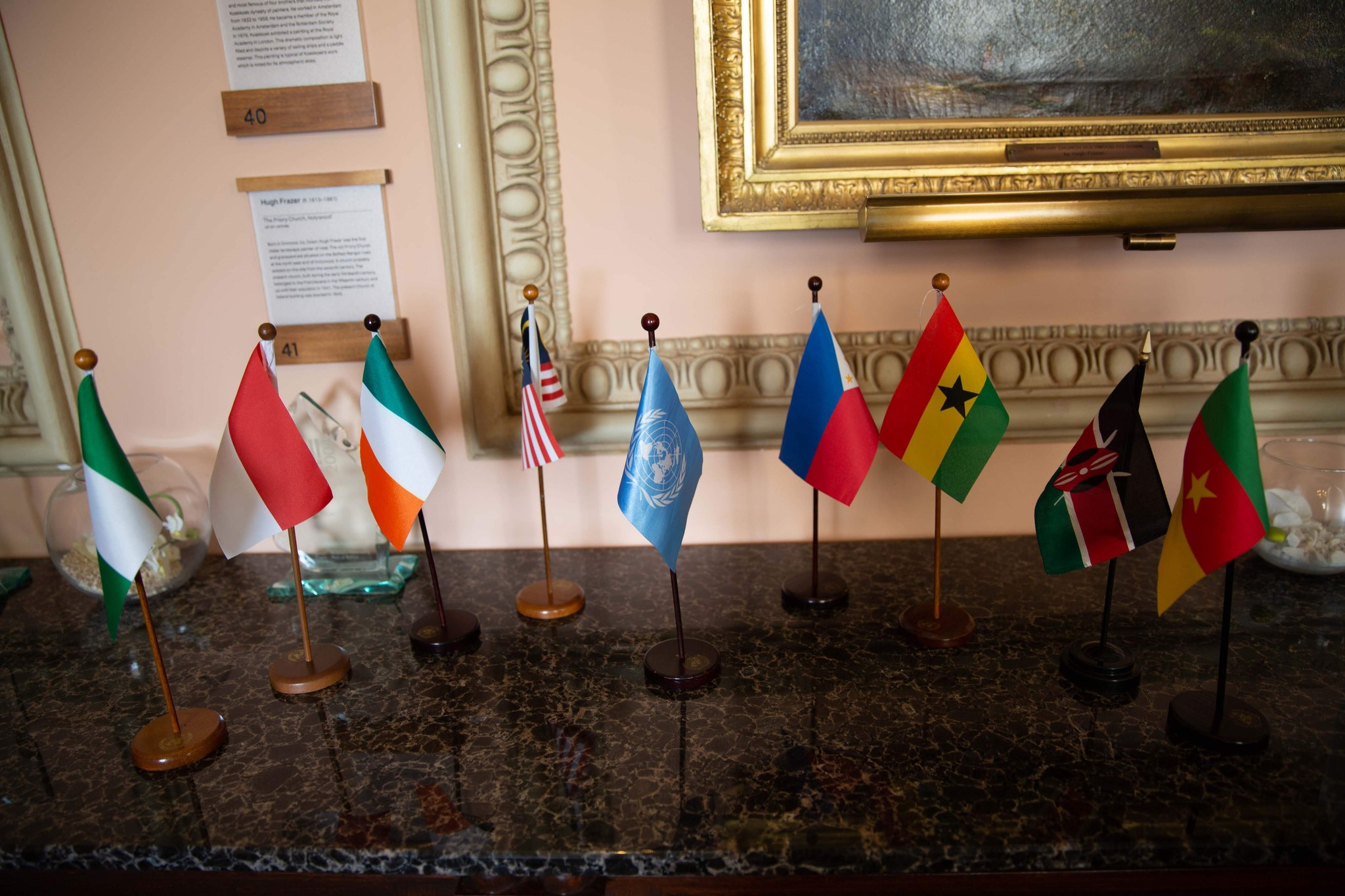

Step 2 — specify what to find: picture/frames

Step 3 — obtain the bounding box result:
[692,0,1344,234]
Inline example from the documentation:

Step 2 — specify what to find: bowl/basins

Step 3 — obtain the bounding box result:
[1250,437,1345,573]
[44,454,209,598]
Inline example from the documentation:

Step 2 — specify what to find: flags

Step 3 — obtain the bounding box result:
[881,293,1011,502]
[359,335,448,555]
[778,305,878,507]
[74,369,167,644]
[519,308,567,472]
[208,344,334,559]
[617,346,705,573]
[1156,364,1271,616]
[1033,361,1172,574]
